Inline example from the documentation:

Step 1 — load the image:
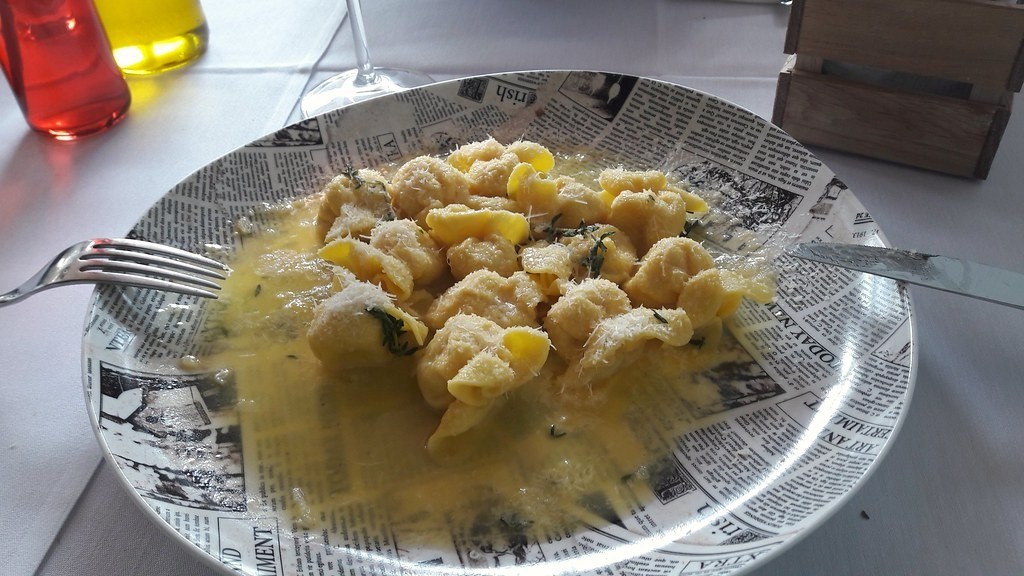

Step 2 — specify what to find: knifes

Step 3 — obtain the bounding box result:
[785,243,1024,308]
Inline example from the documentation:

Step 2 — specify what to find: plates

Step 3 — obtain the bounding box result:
[82,71,917,575]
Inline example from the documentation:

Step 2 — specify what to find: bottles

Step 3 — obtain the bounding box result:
[95,1,208,75]
[1,0,130,142]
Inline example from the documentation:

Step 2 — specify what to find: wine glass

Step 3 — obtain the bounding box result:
[299,0,435,118]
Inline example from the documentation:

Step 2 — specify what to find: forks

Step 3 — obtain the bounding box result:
[0,238,229,306]
